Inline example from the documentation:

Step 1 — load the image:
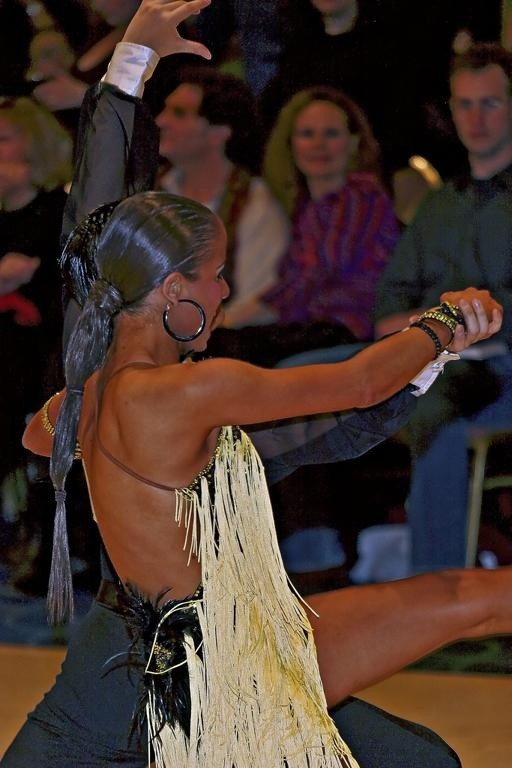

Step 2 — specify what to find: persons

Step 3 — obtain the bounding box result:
[20,188,512,768]
[1,1,511,653]
[0,3,463,768]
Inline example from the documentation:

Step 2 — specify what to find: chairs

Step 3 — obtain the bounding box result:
[396,427,511,571]
[389,155,443,225]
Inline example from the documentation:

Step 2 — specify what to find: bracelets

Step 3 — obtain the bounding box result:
[410,300,468,360]
